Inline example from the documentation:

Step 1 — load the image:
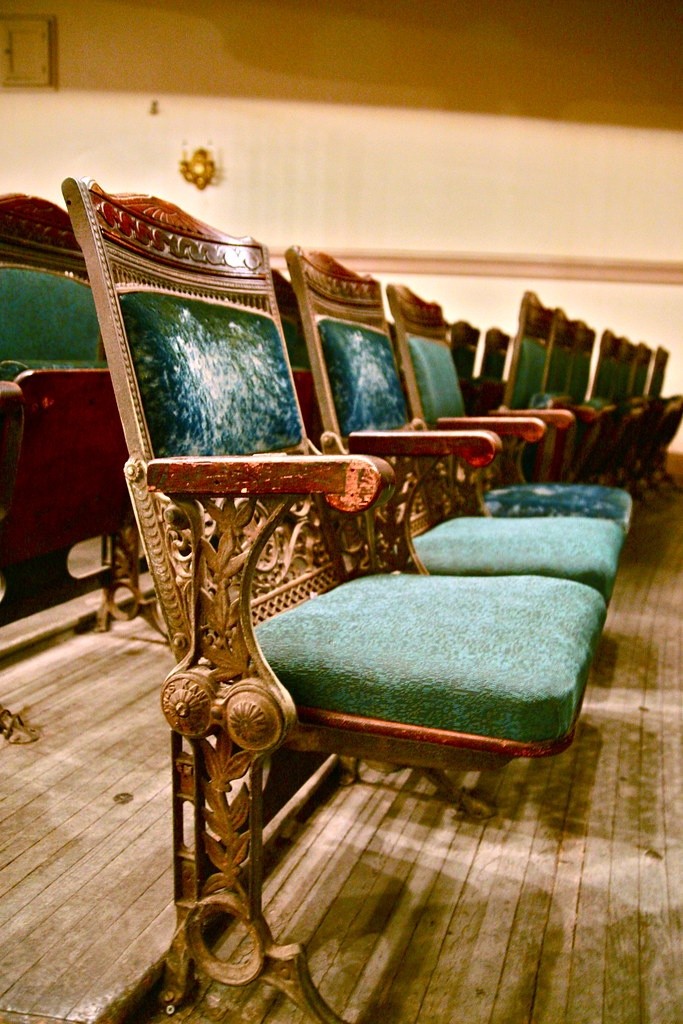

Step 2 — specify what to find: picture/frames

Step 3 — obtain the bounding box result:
[0,12,57,93]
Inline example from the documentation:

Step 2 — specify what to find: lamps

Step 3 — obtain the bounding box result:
[181,139,227,188]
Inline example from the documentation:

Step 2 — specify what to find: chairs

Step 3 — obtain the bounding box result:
[290,246,623,820]
[61,175,606,1024]
[635,341,683,484]
[501,292,594,487]
[385,282,634,537]
[591,329,619,493]
[0,193,174,745]
[267,268,324,455]
[614,337,635,483]
[479,325,511,418]
[569,319,613,484]
[451,320,478,422]
[542,305,600,495]
